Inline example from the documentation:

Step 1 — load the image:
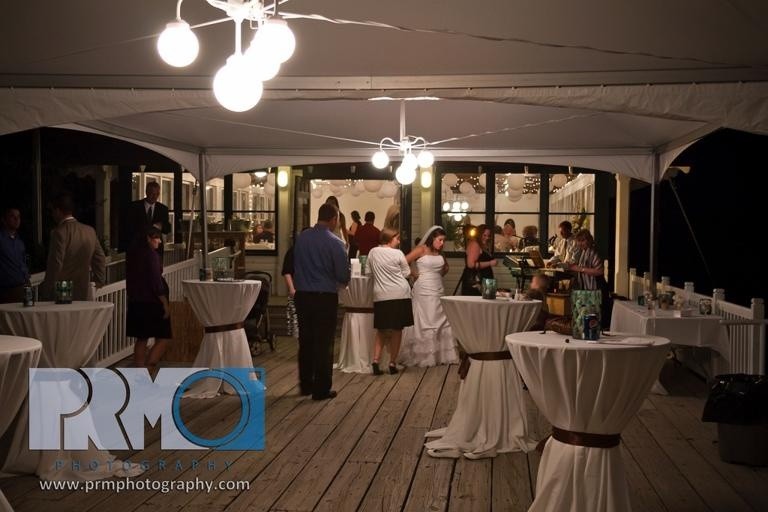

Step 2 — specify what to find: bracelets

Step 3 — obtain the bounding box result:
[583,268,585,273]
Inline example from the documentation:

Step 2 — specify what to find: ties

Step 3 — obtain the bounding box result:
[147,206,152,218]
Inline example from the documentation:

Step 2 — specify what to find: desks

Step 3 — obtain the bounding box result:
[341,276,374,370]
[181,278,262,391]
[0,334,42,368]
[614,299,723,386]
[440,296,542,442]
[0,301,114,468]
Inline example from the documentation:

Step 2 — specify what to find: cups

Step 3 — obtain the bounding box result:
[351,258,359,274]
[353,264,361,277]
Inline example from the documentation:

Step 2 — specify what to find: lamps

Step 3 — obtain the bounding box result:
[156,0,296,113]
[440,195,470,222]
[372,99,434,187]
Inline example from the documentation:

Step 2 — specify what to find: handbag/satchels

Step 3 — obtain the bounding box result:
[464,278,482,296]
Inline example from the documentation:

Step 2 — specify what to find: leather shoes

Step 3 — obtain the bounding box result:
[427,449,459,459]
[312,391,337,401]
[464,448,498,460]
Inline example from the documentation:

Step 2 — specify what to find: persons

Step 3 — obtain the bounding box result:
[546,221,577,268]
[0,203,32,304]
[395,225,460,366]
[460,224,498,296]
[494,218,541,252]
[567,228,611,328]
[129,181,172,275]
[243,214,275,244]
[364,227,415,374]
[38,194,106,301]
[126,225,173,377]
[281,227,314,339]
[524,274,550,330]
[326,196,380,258]
[294,203,351,401]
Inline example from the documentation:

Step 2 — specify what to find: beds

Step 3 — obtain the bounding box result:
[505,331,671,512]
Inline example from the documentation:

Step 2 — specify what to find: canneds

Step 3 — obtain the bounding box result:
[24,287,35,308]
[584,314,598,340]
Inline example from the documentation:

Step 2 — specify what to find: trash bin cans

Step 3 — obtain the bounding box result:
[701,373,767,467]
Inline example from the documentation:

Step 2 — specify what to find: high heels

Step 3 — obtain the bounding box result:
[372,358,381,375]
[389,361,398,374]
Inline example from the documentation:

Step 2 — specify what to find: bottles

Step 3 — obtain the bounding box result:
[647,299,656,317]
[359,255,367,275]
